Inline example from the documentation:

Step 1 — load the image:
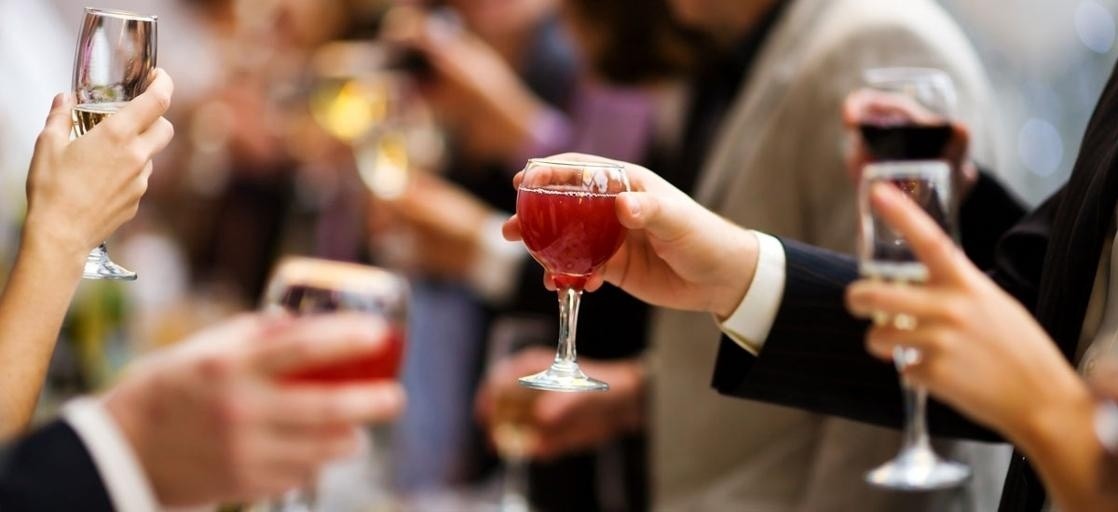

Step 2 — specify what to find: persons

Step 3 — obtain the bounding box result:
[1,0,1118,510]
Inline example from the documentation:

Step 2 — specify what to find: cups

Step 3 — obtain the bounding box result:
[852,69,959,163]
[262,254,411,386]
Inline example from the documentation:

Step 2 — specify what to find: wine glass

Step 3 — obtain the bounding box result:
[509,154,636,397]
[69,5,160,282]
[858,161,976,493]
